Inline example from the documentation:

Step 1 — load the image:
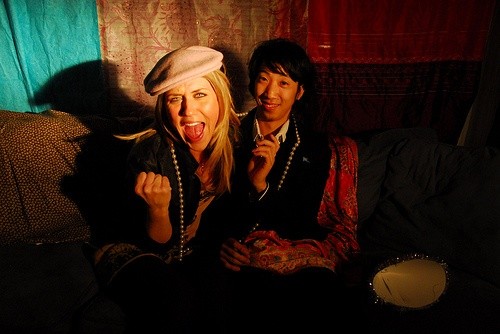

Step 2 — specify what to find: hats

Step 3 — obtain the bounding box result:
[143,46,224,98]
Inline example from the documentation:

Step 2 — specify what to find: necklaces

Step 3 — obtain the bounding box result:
[168,139,191,263]
[274,112,300,190]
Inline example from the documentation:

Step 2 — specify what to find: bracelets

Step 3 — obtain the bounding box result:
[247,182,269,202]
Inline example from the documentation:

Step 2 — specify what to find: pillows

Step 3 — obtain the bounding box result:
[1,108,155,249]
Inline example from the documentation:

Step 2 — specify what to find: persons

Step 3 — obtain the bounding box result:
[91,46,277,333]
[219,39,359,328]
